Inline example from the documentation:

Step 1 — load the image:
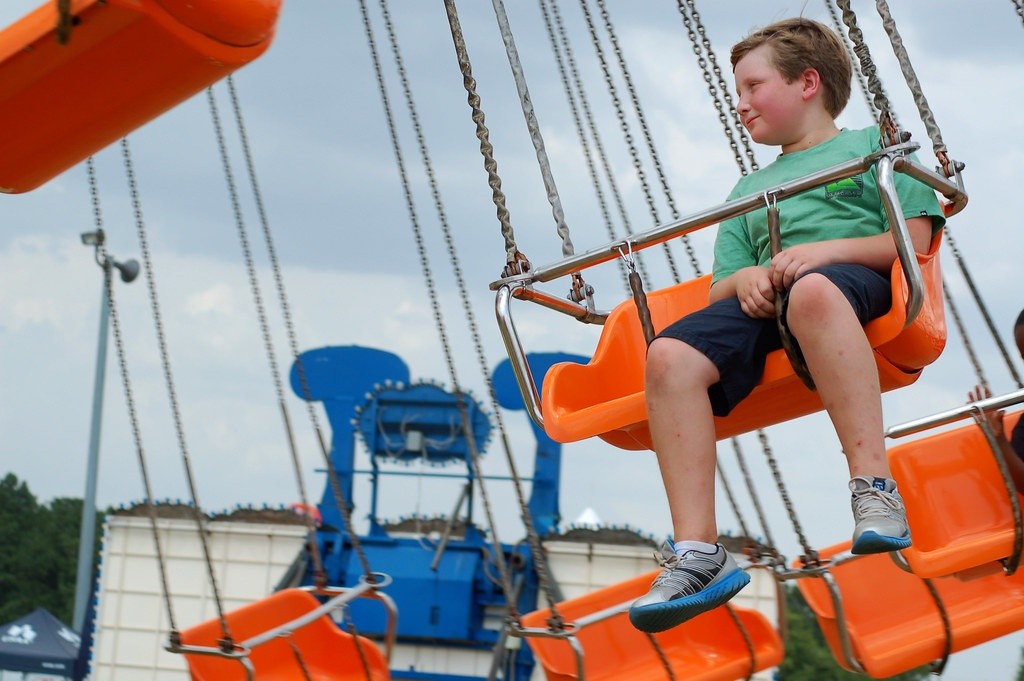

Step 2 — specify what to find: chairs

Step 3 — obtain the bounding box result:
[882,410,1024,578]
[795,532,1024,679]
[181,589,395,681]
[515,576,786,681]
[0,0,286,200]
[533,195,957,461]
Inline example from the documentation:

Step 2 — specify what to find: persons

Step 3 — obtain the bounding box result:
[628,0,946,634]
[969,310,1024,495]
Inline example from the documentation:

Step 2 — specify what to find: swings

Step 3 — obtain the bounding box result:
[89,0,1023,678]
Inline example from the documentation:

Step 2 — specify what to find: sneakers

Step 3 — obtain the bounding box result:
[848,475,913,555]
[630,542,751,633]
